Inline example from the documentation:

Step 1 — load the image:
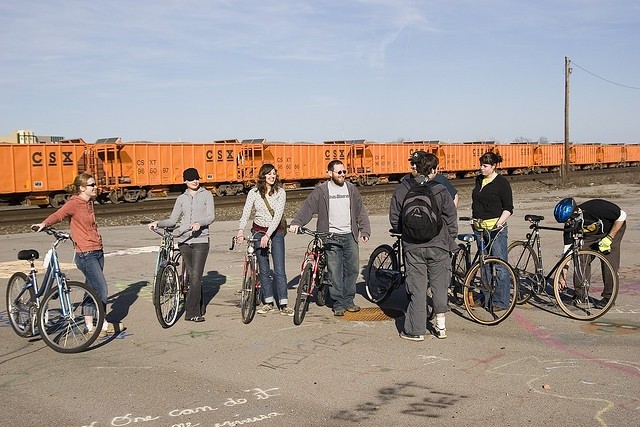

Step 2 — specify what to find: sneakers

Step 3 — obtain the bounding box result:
[101,321,124,332]
[256,303,274,314]
[83,326,109,337]
[186,315,205,322]
[279,307,297,316]
[595,299,613,309]
[431,324,447,339]
[486,305,506,312]
[568,295,591,305]
[345,304,361,311]
[333,306,345,317]
[399,331,425,341]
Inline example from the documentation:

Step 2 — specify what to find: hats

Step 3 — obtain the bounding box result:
[409,152,428,161]
[183,168,201,180]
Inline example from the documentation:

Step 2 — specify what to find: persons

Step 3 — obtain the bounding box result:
[148,168,215,321]
[554,197,627,308]
[389,154,459,340]
[472,152,514,311]
[289,160,372,315]
[406,153,458,211]
[236,163,296,316]
[31,174,122,334]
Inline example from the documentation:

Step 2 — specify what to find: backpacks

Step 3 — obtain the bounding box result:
[399,178,443,243]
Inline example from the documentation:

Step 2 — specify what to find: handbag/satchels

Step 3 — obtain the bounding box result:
[278,218,288,233]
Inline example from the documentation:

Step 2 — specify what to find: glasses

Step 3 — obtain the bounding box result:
[410,162,419,164]
[87,183,97,187]
[187,180,199,181]
[332,169,348,175]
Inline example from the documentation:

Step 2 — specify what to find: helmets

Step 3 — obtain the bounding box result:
[553,198,577,223]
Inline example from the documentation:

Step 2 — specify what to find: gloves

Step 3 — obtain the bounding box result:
[597,235,613,254]
[553,278,569,292]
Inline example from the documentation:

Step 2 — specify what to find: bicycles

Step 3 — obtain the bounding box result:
[229,236,273,324]
[366,228,436,321]
[286,224,336,325]
[449,216,518,325]
[150,220,194,328]
[6,224,104,353]
[507,208,619,321]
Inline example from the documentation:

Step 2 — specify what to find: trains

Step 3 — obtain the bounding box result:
[0,136,640,207]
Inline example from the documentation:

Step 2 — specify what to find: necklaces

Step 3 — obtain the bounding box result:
[484,173,496,183]
